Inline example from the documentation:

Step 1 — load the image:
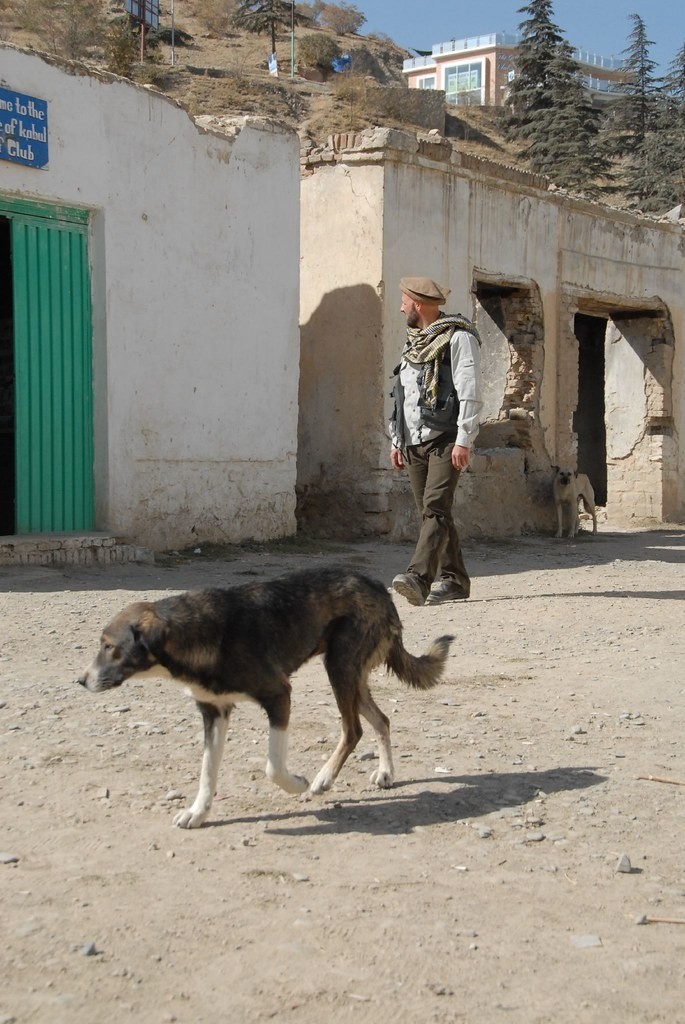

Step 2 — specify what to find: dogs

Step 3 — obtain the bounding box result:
[79,565,456,836]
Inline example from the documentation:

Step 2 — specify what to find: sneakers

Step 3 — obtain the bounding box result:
[428,581,470,601]
[391,572,432,605]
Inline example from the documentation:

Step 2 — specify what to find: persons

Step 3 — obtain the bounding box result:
[389,277,484,606]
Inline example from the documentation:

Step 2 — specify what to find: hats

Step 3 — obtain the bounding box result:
[400,277,453,306]
